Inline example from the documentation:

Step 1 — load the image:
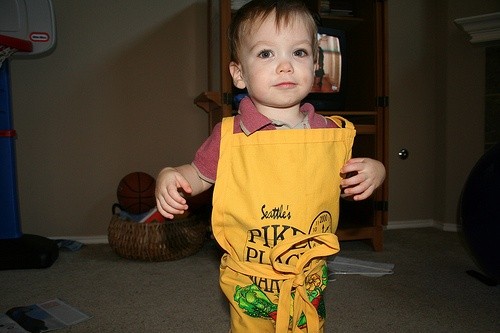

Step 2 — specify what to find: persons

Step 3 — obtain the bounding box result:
[154,0,386,332]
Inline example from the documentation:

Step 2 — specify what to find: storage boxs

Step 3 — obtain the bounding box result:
[107,214,209,264]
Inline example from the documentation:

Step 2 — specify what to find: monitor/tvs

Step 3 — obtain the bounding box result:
[300,27,348,111]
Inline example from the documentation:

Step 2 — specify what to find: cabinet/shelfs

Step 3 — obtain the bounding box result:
[193,0,390,255]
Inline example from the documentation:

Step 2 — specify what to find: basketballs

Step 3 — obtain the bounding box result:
[116,171,157,215]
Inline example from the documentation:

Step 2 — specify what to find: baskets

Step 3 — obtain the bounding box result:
[108,213,206,261]
